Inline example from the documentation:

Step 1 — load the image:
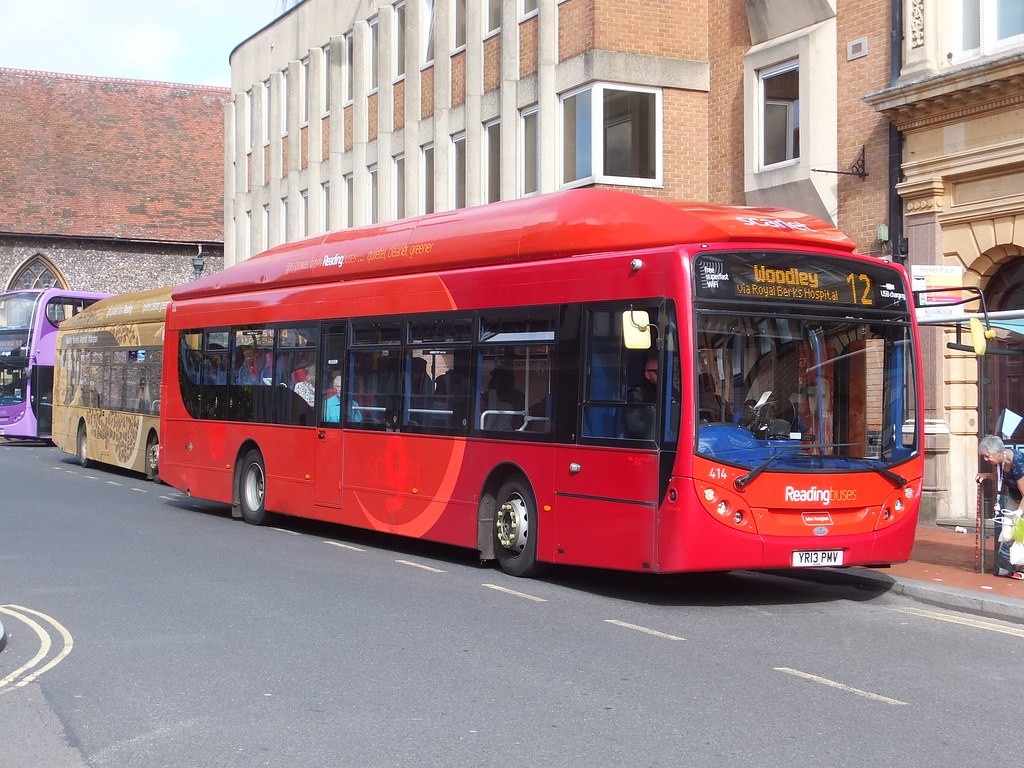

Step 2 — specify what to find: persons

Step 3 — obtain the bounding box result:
[975,434,1024,580]
[190,342,814,451]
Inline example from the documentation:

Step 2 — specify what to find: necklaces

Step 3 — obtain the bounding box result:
[1000,449,1007,480]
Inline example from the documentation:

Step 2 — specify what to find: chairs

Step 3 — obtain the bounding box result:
[190,358,548,435]
[761,418,793,440]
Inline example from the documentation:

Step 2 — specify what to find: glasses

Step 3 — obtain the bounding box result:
[645,369,657,375]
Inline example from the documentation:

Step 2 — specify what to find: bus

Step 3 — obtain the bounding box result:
[51,287,181,484]
[0,288,116,447]
[158,189,995,578]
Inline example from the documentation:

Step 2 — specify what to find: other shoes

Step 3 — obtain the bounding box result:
[1010,571,1024,580]
